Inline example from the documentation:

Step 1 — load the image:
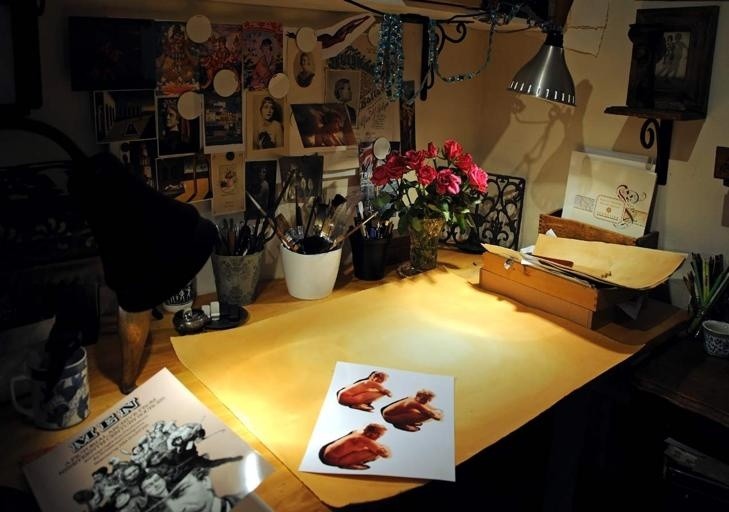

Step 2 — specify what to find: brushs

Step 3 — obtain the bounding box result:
[210,166,395,255]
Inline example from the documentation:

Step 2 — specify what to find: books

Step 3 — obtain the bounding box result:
[558,147,658,238]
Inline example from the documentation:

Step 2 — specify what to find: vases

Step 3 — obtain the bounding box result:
[406,218,446,271]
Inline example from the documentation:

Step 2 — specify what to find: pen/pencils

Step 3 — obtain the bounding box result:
[350,200,394,241]
[215,215,260,256]
[683,252,729,339]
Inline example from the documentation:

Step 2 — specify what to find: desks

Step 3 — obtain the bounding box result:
[1,242,691,512]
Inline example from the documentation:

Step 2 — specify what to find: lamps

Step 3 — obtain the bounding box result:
[506,31,576,108]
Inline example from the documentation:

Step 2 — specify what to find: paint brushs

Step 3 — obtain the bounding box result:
[246,190,378,254]
[261,169,295,234]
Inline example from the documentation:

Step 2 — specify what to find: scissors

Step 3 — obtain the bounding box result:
[253,214,277,253]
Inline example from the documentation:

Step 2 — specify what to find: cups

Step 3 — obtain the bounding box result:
[280,239,342,300]
[211,247,265,307]
[348,235,392,281]
[10,340,90,431]
[701,319,729,357]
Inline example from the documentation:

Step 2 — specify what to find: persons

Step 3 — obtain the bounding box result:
[295,52,315,87]
[319,422,389,469]
[380,389,443,433]
[159,105,187,157]
[315,113,343,148]
[207,36,233,81]
[337,371,392,411]
[88,420,244,512]
[253,97,283,148]
[334,78,356,123]
[252,37,278,91]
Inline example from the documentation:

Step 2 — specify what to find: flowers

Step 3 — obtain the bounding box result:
[370,139,488,235]
